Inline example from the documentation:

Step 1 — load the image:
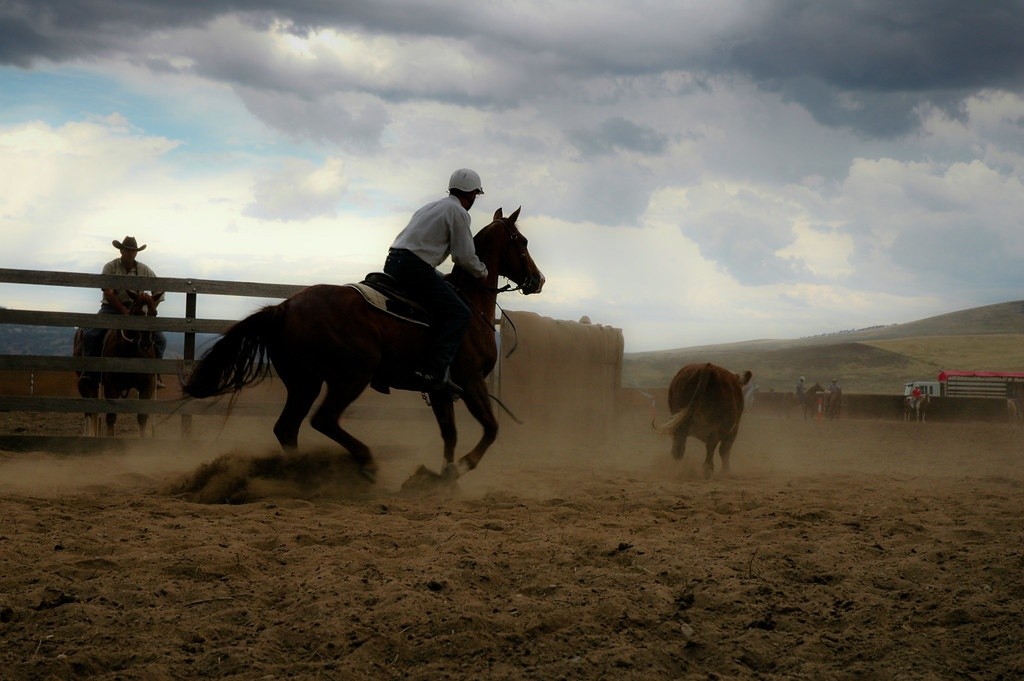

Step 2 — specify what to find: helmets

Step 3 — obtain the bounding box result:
[448,169,485,194]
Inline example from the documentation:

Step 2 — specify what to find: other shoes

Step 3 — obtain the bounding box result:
[158,382,166,388]
[80,372,92,379]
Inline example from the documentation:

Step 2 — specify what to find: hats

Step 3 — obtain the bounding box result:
[112,236,147,251]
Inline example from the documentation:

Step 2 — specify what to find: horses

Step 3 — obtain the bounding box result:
[149,206,547,484]
[71,289,167,438]
[903,396,930,424]
[782,383,824,421]
[652,362,753,482]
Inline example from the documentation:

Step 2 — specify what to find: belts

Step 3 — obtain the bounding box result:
[391,250,408,254]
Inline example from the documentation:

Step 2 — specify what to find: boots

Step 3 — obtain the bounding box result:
[433,343,464,395]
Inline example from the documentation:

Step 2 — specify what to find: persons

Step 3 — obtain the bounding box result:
[828,379,841,420]
[797,376,810,405]
[87,236,172,391]
[939,369,949,400]
[912,385,921,410]
[385,167,490,402]
